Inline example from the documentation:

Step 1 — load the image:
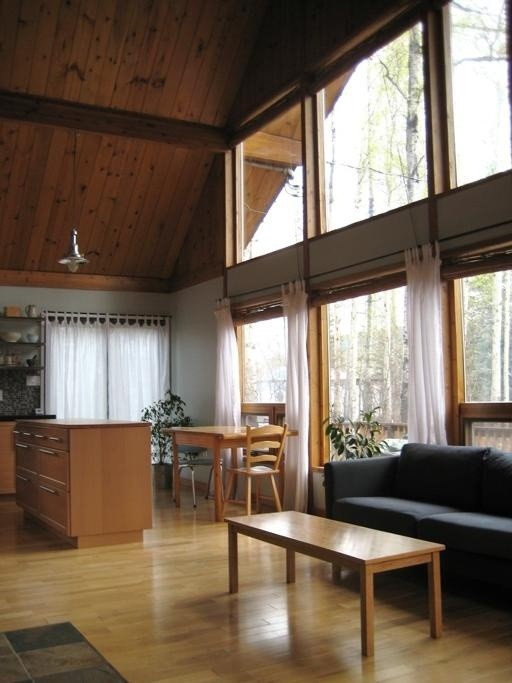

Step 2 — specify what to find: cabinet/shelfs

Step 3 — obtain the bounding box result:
[9,416,153,548]
[2,314,56,499]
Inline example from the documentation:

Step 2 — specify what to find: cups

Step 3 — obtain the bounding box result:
[0,354,16,366]
[26,359,34,367]
[25,304,37,317]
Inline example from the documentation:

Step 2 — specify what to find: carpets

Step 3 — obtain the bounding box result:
[1,617,128,681]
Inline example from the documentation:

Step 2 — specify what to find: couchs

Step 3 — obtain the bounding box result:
[322,443,510,608]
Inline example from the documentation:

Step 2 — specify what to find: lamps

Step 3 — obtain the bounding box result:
[56,63,91,272]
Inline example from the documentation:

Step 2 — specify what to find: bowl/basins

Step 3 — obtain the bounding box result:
[27,335,39,342]
[0,332,21,343]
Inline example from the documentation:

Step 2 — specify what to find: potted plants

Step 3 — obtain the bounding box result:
[139,388,194,490]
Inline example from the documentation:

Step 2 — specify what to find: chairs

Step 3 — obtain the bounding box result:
[217,424,292,525]
[177,424,223,507]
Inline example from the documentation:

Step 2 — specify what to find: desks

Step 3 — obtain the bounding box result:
[159,424,298,520]
